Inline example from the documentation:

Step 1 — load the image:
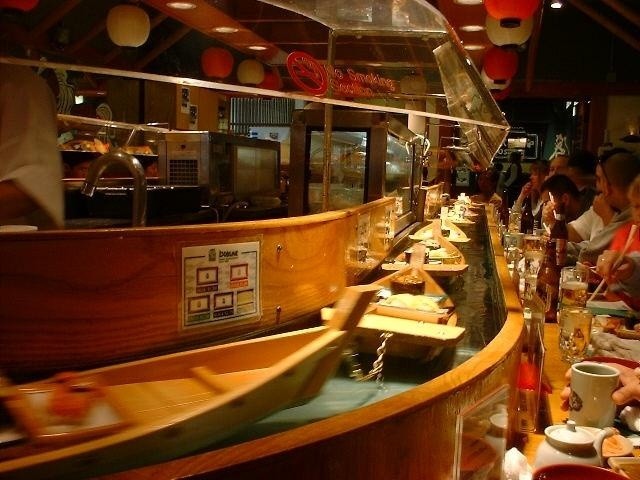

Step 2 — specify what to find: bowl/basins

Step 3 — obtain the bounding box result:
[461,435,497,480]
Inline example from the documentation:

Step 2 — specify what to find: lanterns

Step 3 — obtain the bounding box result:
[237,57,265,91]
[482,0,540,28]
[485,14,536,48]
[484,47,520,85]
[257,65,283,101]
[480,71,511,92]
[106,4,152,50]
[202,44,234,86]
[489,87,512,101]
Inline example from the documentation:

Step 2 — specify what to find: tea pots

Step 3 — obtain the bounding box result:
[535,422,615,469]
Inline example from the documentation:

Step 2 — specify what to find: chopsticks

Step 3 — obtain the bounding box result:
[585,224,638,306]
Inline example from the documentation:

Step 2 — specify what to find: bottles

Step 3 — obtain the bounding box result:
[535,240,559,324]
[499,190,509,230]
[551,206,568,268]
[521,196,534,234]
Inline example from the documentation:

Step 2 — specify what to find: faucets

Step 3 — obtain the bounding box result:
[221,197,250,220]
[198,204,219,222]
[80,149,150,227]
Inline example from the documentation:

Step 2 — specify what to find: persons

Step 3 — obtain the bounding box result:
[488,135,640,413]
[0,58,65,231]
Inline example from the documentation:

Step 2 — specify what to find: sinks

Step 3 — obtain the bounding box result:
[232,194,281,219]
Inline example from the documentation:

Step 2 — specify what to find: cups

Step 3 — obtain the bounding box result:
[560,306,592,364]
[571,362,619,427]
[559,267,588,309]
